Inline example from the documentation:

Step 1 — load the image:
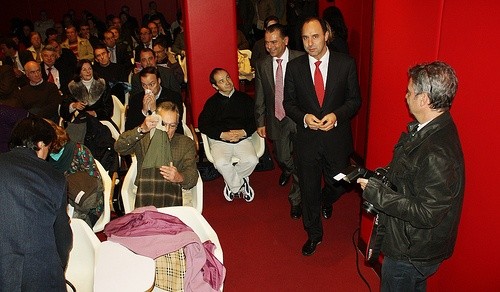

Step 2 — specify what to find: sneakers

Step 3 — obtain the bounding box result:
[223,181,235,202]
[240,176,254,202]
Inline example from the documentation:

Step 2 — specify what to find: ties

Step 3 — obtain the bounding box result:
[48,68,54,82]
[274,59,285,121]
[12,57,18,69]
[109,48,114,62]
[146,44,149,48]
[314,61,324,109]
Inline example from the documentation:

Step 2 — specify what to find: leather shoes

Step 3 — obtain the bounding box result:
[302,239,321,255]
[291,205,301,219]
[279,172,289,186]
[322,202,332,219]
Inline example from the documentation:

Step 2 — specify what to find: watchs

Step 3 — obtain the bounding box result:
[334,121,338,127]
[138,125,149,135]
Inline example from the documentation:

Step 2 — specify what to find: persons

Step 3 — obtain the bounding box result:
[0,0,199,292]
[237,0,362,256]
[197,67,266,203]
[356,61,465,292]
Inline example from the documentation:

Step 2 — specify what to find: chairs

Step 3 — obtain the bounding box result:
[200,131,265,164]
[113,205,224,292]
[93,92,204,232]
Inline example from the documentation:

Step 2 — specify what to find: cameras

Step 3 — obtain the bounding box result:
[341,164,398,214]
